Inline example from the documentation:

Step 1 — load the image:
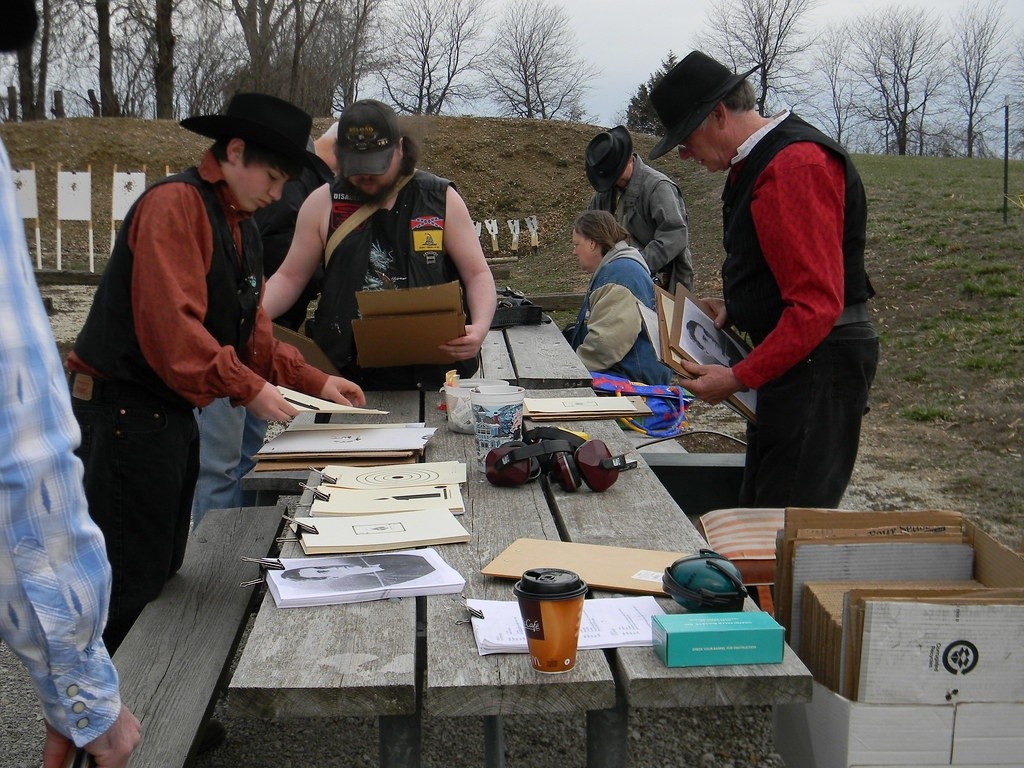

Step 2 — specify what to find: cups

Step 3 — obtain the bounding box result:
[513,569,588,674]
[470,385,526,473]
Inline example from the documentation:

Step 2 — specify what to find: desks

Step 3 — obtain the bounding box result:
[462,307,595,389]
[226,390,816,768]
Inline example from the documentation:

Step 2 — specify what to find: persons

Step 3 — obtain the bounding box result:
[253,98,499,392]
[585,125,696,297]
[573,210,672,388]
[60,93,367,757]
[647,50,880,510]
[0,143,141,768]
[686,320,744,368]
[192,119,340,532]
[280,555,436,581]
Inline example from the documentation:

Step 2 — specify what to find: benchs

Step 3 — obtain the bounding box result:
[41,502,287,768]
[620,428,690,453]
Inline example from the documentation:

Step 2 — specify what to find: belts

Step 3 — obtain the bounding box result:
[832,302,872,328]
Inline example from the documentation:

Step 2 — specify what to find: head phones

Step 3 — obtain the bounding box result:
[485,426,627,492]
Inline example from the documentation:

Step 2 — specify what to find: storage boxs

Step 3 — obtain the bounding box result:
[773,504,1023,767]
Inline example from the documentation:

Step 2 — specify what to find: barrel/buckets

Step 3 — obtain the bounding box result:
[439,378,509,433]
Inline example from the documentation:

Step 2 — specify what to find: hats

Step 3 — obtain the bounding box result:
[180,93,336,183]
[649,50,769,162]
[584,125,632,193]
[335,100,402,177]
